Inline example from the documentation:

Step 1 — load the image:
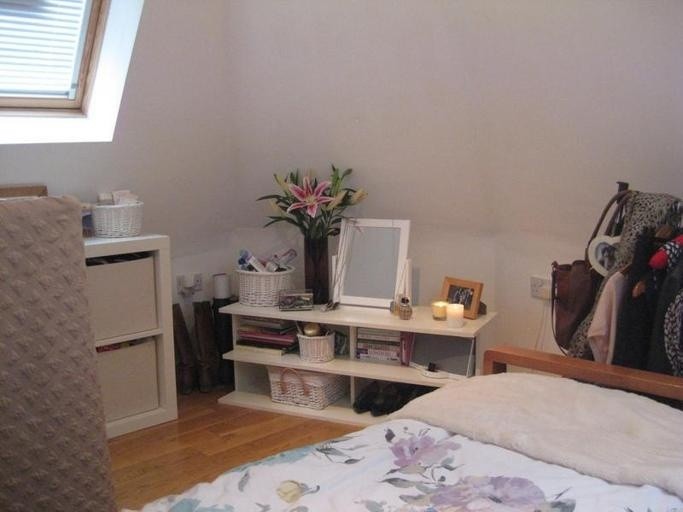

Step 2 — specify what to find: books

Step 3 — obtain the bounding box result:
[355,327,400,363]
[235,317,298,359]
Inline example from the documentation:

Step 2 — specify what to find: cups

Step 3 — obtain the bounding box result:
[445,303,464,328]
[431,301,448,321]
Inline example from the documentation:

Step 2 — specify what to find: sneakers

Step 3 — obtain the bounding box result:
[352,381,424,416]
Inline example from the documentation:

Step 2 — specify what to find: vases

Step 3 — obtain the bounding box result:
[303,236,330,305]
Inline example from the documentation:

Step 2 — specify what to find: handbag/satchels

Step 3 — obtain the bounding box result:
[549,260,602,350]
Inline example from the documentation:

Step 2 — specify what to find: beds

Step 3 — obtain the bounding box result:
[123,343,683,512]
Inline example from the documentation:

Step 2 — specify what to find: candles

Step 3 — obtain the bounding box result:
[446,302,464,328]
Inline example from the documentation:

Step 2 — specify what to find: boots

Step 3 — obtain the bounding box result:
[172,302,214,394]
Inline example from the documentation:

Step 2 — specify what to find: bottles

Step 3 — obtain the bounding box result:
[398,297,412,320]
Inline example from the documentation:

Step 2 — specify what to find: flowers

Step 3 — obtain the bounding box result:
[254,164,369,239]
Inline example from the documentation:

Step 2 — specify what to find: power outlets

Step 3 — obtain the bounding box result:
[529,275,556,299]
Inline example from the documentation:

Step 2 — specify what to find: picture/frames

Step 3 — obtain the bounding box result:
[333,216,410,308]
[438,276,483,320]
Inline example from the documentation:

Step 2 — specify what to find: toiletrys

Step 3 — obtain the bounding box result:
[236,246,298,272]
[399,332,416,366]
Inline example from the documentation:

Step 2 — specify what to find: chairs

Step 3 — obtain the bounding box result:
[0,194,123,512]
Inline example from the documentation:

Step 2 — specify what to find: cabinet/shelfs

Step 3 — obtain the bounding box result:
[218,297,498,426]
[82,236,178,442]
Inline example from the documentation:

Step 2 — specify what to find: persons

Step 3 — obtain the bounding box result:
[449,288,473,308]
